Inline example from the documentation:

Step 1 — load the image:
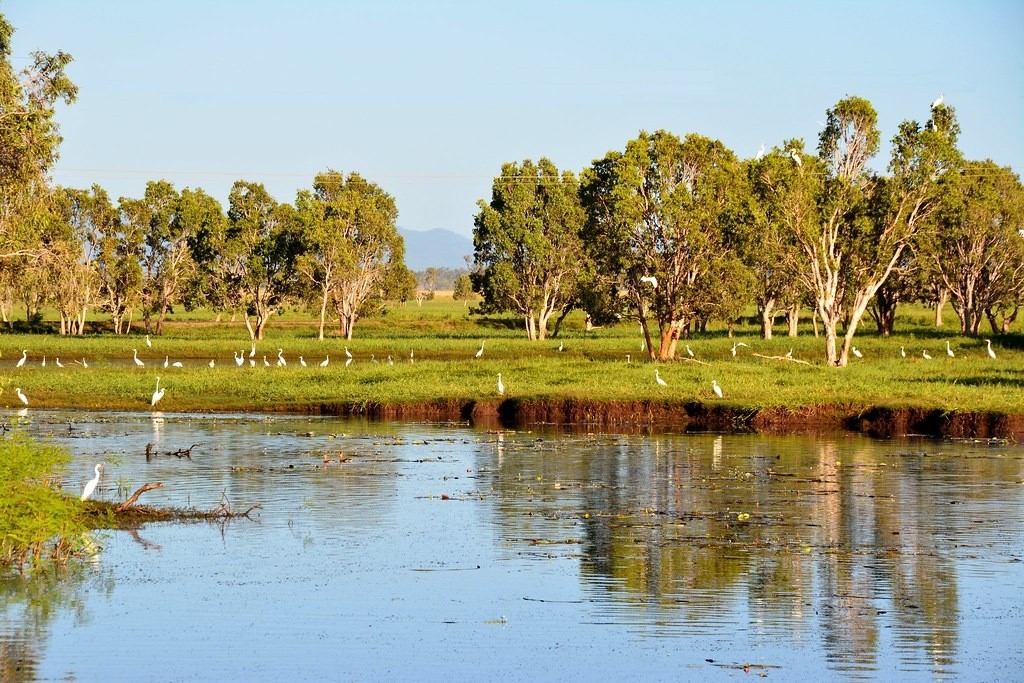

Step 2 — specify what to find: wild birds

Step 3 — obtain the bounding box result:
[208,342,306,369]
[559,341,564,351]
[850,341,954,360]
[686,342,748,398]
[370,348,413,360]
[80,464,102,502]
[320,346,352,367]
[15,350,88,405]
[984,339,996,359]
[655,370,667,386]
[785,348,794,357]
[345,358,352,367]
[497,373,505,395]
[131,335,183,406]
[474,340,486,359]
[626,341,644,364]
